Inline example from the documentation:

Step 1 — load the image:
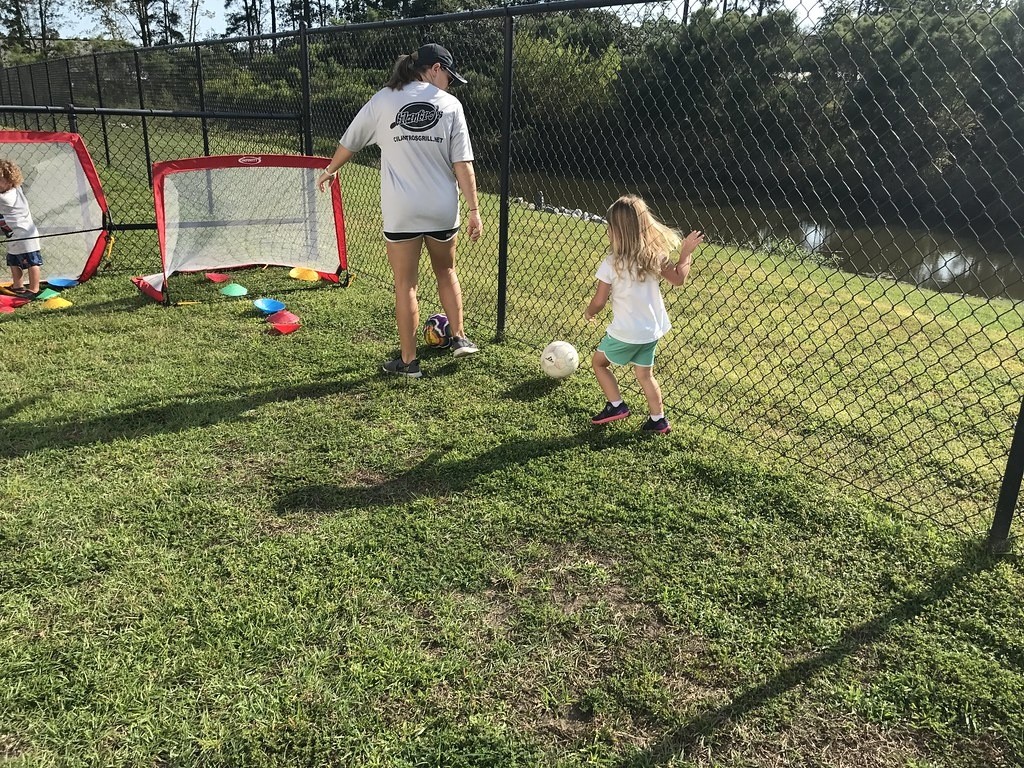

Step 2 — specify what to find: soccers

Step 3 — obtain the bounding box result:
[540,341,580,379]
[425,312,455,349]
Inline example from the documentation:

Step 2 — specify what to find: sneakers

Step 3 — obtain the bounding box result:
[383,357,422,378]
[452,336,480,358]
[641,416,669,432]
[591,400,630,424]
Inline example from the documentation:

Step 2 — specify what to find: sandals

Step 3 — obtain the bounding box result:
[0,284,26,296]
[15,289,43,299]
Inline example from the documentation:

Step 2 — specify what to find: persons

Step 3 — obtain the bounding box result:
[318,42,483,378]
[0,159,43,299]
[584,194,705,431]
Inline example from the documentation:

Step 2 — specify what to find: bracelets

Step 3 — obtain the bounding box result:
[470,208,479,212]
[325,166,337,176]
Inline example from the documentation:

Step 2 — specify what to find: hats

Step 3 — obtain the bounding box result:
[414,43,468,87]
